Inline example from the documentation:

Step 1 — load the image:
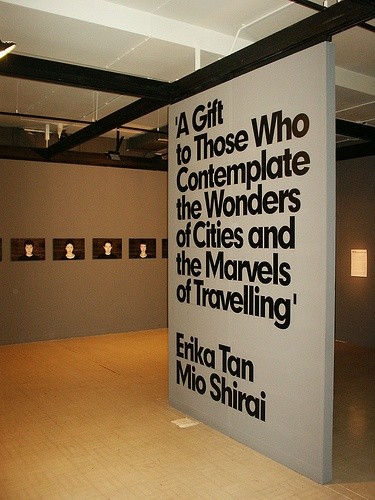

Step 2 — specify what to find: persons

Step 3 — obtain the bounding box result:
[96,241,118,259]
[58,240,80,261]
[135,241,152,258]
[17,239,41,261]
